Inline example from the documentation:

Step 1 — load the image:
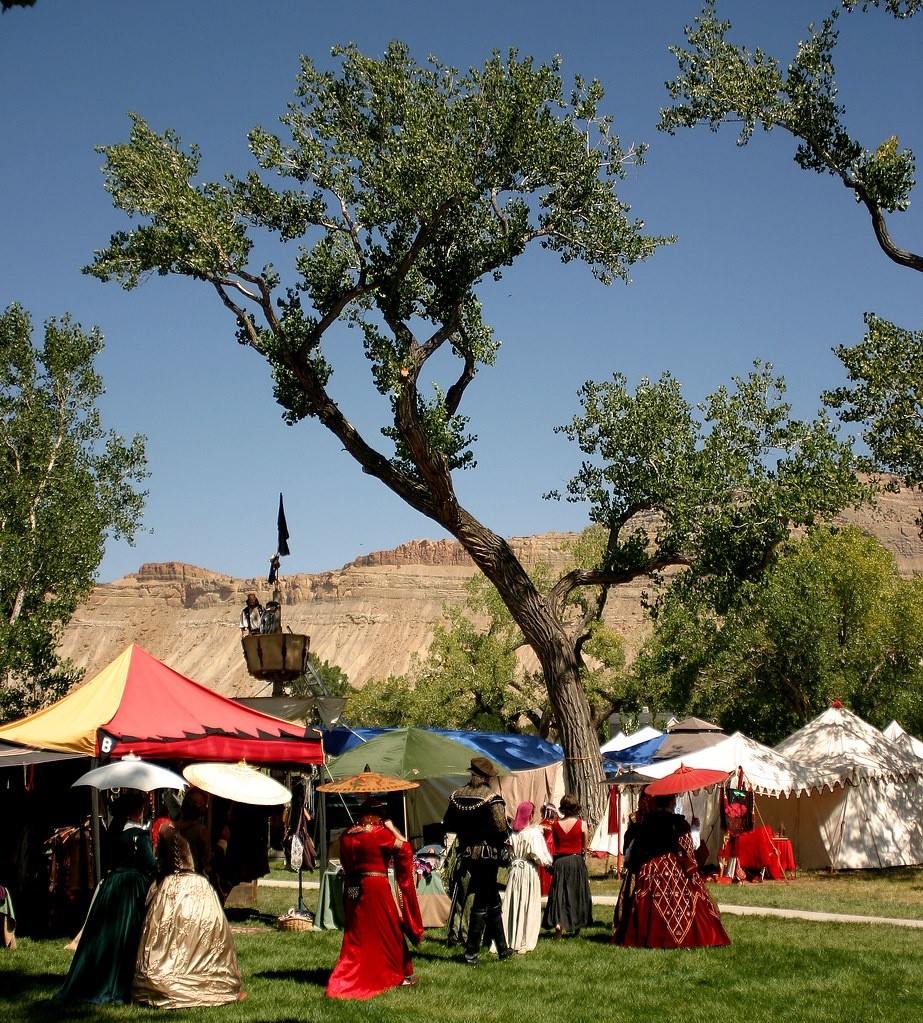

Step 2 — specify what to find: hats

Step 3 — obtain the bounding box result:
[466,757,499,778]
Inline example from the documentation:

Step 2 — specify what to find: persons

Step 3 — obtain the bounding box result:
[443,756,516,966]
[326,795,424,999]
[610,783,732,949]
[239,593,266,639]
[489,794,592,954]
[54,786,242,1009]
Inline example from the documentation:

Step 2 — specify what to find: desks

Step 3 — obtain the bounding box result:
[717,834,792,881]
[315,858,445,932]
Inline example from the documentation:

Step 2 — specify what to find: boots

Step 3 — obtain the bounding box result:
[464,907,487,964]
[487,903,513,960]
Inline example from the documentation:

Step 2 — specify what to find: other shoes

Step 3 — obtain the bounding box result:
[555,925,562,939]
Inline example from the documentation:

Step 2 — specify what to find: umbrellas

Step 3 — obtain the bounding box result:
[71,751,190,827]
[315,763,420,797]
[645,763,730,818]
[183,760,293,806]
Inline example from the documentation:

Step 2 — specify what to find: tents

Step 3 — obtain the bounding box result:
[323,699,923,872]
[0,643,329,884]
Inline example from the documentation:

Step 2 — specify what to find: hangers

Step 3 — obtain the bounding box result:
[45,819,90,846]
